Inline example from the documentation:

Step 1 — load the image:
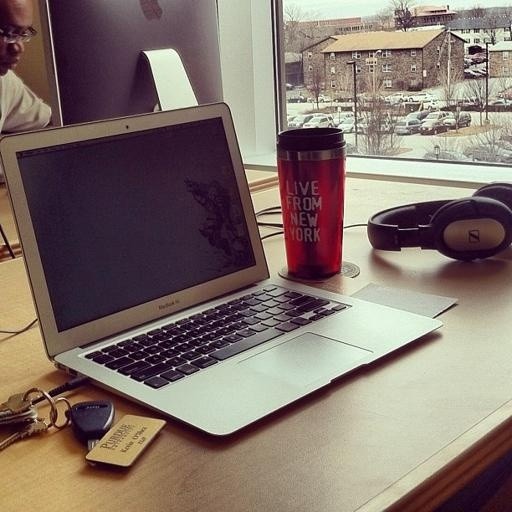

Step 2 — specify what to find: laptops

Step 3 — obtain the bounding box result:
[2,100,444,438]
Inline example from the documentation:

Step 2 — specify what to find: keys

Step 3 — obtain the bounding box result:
[0,393,38,425]
[69,400,115,467]
[0,419,49,451]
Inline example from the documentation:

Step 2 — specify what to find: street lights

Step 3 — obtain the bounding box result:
[372,50,383,92]
[346,60,359,148]
[483,37,493,119]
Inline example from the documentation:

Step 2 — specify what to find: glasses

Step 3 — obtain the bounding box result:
[0,28,38,45]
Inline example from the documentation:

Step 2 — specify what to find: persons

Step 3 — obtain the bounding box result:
[0,1,54,141]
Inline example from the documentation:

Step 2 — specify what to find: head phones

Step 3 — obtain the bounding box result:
[367,181,512,261]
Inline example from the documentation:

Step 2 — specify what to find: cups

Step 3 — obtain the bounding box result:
[275,126,346,280]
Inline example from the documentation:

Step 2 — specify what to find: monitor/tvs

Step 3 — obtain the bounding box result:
[39,0,226,126]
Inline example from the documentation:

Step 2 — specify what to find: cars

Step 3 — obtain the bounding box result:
[421,134,511,164]
[287,88,512,136]
[284,83,293,91]
[460,50,490,80]
[306,84,324,92]
